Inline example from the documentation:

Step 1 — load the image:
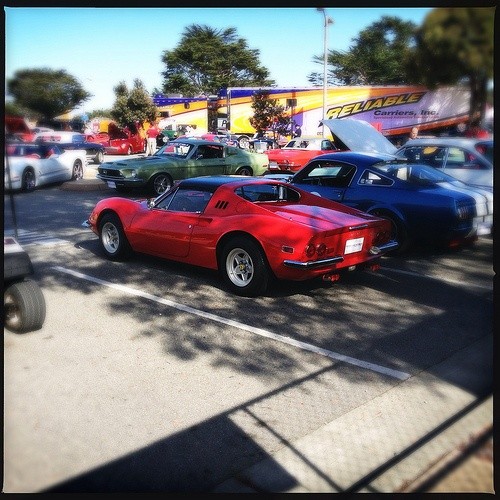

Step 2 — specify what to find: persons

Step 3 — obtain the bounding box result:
[402,127,422,161]
[145,122,158,157]
[292,124,302,139]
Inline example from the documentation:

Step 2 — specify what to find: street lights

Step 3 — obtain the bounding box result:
[315,8,335,148]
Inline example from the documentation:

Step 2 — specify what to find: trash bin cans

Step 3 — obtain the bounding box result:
[253,140,270,153]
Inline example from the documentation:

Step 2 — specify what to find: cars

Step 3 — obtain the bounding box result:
[6,127,105,166]
[86,174,397,299]
[99,137,268,197]
[4,236,46,335]
[102,133,146,155]
[256,153,494,252]
[3,141,87,191]
[321,117,493,194]
[262,137,336,174]
[186,133,281,149]
